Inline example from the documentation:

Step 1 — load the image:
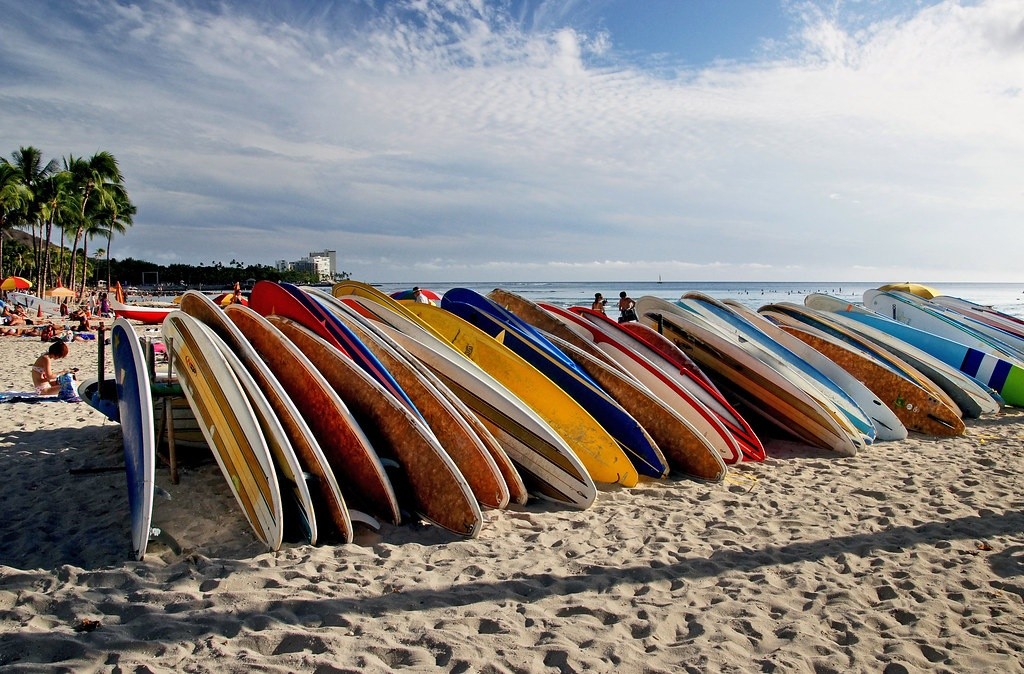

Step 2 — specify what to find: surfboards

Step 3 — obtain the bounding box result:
[110,316,157,563]
[161,279,1024,549]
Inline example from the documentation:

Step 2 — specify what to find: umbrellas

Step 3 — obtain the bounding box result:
[211,293,250,308]
[388,290,441,302]
[0,276,33,290]
[115,282,125,319]
[877,282,943,301]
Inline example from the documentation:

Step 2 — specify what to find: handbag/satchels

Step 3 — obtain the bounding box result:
[621,308,638,322]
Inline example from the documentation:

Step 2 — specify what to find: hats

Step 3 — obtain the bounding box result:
[91,291,96,294]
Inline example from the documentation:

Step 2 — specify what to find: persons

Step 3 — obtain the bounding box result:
[412,287,437,307]
[0,275,111,342]
[32,341,79,395]
[592,293,608,317]
[618,291,639,324]
[230,279,243,305]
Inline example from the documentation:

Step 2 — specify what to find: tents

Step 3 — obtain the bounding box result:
[45,287,75,297]
